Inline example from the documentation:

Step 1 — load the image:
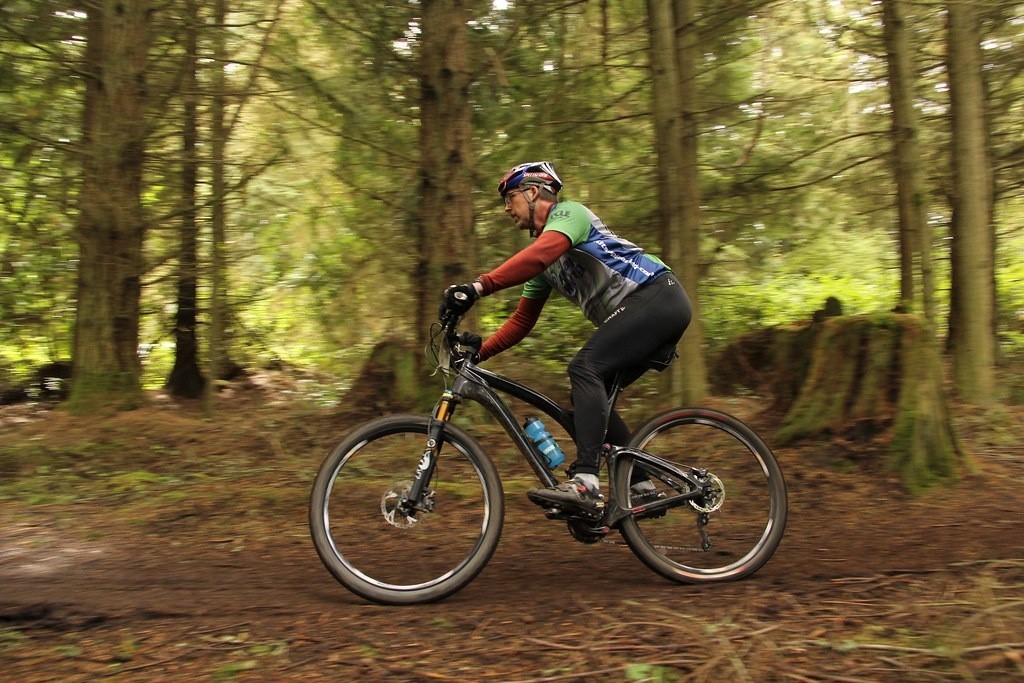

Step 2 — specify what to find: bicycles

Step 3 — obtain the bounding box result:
[308,286,788,605]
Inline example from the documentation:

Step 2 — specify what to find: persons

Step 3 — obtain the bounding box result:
[441,161,694,519]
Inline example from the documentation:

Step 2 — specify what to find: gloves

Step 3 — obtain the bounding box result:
[442,283,480,317]
[451,331,481,368]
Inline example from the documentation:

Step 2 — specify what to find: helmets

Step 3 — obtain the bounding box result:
[498,160,562,195]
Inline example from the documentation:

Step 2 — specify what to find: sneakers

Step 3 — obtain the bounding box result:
[525,478,606,519]
[631,490,667,519]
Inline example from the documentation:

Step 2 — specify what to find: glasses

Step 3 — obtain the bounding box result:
[504,186,531,203]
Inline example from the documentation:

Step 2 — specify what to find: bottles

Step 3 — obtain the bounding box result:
[523,416,566,468]
